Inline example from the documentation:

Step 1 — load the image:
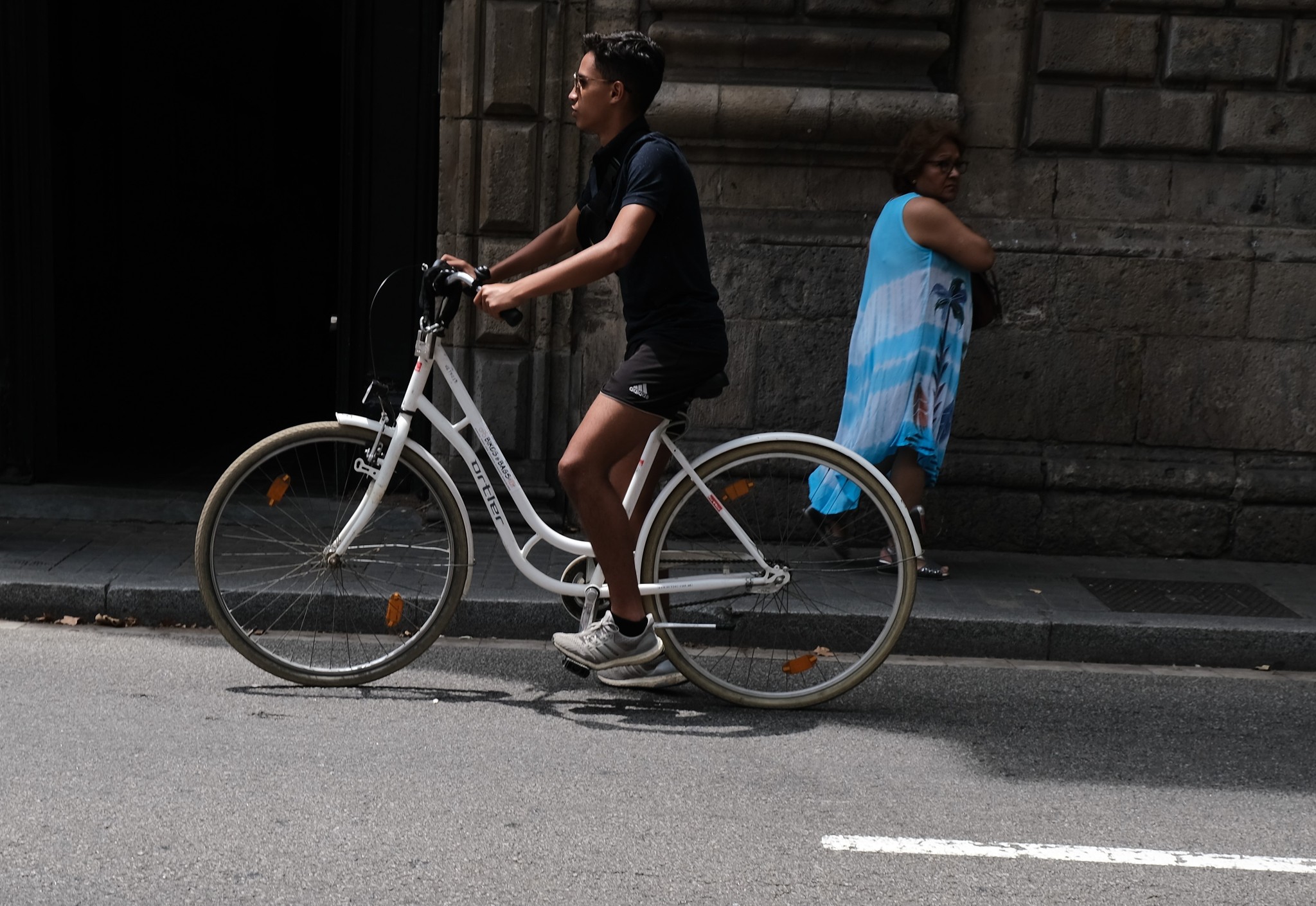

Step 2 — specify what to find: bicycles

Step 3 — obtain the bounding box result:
[193,255,926,711]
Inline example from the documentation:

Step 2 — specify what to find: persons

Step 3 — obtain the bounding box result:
[440,32,731,687]
[801,123,995,580]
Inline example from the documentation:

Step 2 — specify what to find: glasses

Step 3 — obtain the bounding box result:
[574,72,611,93]
[925,159,969,175]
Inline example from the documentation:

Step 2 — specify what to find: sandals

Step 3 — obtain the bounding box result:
[803,504,855,563]
[877,544,951,581]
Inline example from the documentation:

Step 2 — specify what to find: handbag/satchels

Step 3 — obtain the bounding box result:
[971,268,1003,332]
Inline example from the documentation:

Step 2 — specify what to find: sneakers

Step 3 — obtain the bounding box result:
[552,610,664,670]
[597,654,689,688]
